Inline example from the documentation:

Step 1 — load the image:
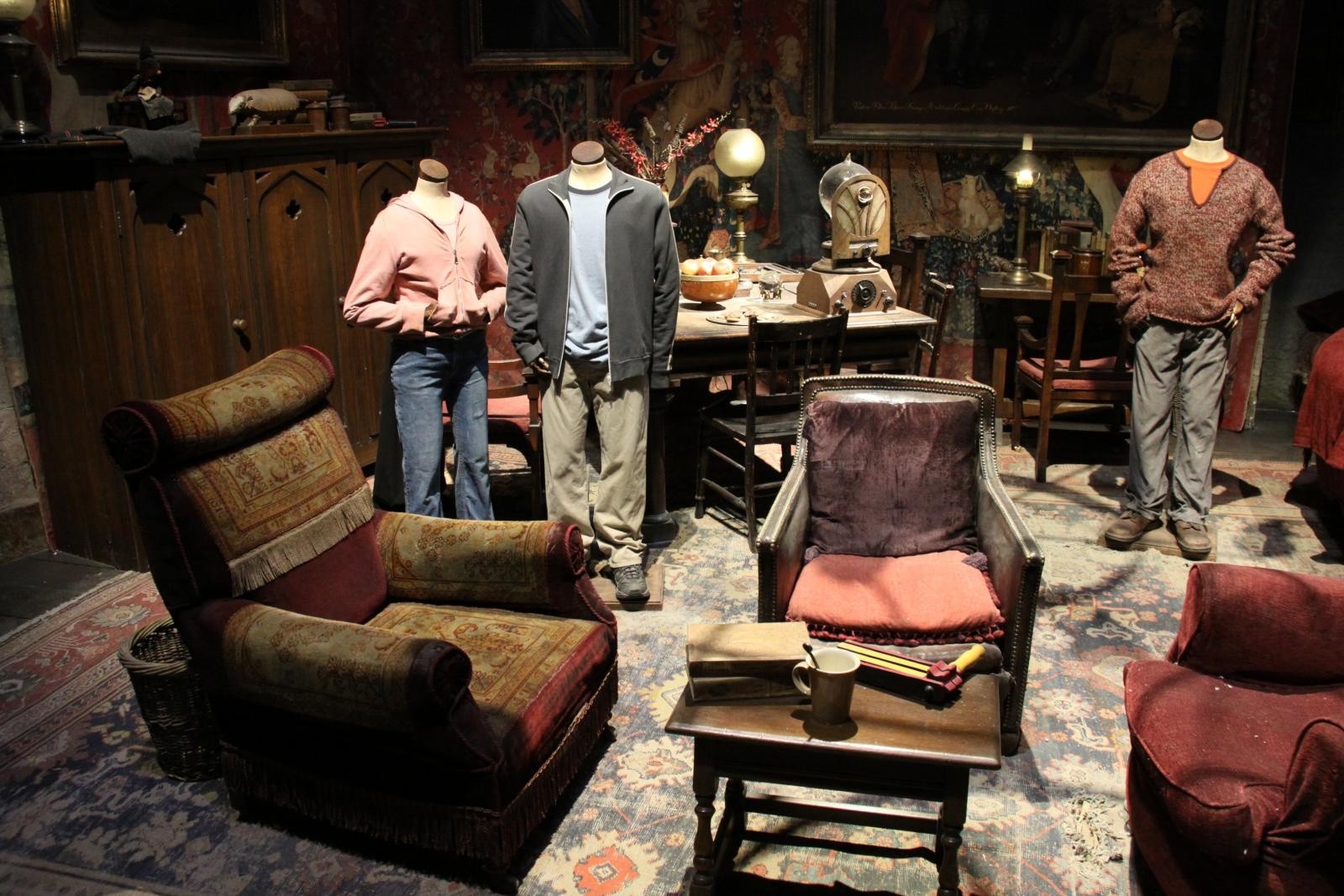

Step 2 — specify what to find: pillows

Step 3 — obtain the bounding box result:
[801,398,989,571]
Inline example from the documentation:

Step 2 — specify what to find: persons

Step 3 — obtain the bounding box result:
[1104,119,1296,556]
[344,158,509,520]
[503,142,681,602]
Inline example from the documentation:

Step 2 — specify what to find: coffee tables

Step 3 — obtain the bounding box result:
[661,673,1004,896]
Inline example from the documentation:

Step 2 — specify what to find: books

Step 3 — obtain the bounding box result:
[682,622,813,704]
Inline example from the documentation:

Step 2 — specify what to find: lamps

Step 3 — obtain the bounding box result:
[1001,133,1051,284]
[713,117,765,263]
[0,0,49,144]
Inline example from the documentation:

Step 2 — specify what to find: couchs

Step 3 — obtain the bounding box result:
[1121,561,1344,896]
[100,344,622,892]
[756,371,1045,758]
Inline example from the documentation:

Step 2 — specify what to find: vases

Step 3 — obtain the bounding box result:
[644,178,669,208]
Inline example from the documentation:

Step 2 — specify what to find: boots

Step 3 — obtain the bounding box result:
[1168,517,1212,553]
[1105,509,1163,542]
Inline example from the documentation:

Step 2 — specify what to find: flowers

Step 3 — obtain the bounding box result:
[589,106,733,178]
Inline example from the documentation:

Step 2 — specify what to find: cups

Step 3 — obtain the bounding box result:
[790,648,860,723]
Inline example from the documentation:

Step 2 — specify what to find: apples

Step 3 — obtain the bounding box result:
[681,258,737,276]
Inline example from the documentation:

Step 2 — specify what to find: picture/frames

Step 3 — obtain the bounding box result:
[800,0,1258,157]
[46,0,295,75]
[461,1,641,74]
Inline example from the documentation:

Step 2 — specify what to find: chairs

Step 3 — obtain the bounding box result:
[914,270,955,378]
[1010,273,1134,482]
[437,351,547,521]
[855,231,931,376]
[690,306,850,556]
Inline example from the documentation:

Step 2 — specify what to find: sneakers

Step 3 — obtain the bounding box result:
[611,564,650,601]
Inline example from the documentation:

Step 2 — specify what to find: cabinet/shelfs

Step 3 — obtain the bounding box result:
[240,119,452,466]
[0,134,248,575]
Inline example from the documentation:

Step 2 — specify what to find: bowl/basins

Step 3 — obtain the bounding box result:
[678,273,739,302]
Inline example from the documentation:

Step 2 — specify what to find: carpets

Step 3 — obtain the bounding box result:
[0,458,1344,896]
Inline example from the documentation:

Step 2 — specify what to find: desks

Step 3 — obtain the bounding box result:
[639,272,939,544]
[975,272,1127,446]
